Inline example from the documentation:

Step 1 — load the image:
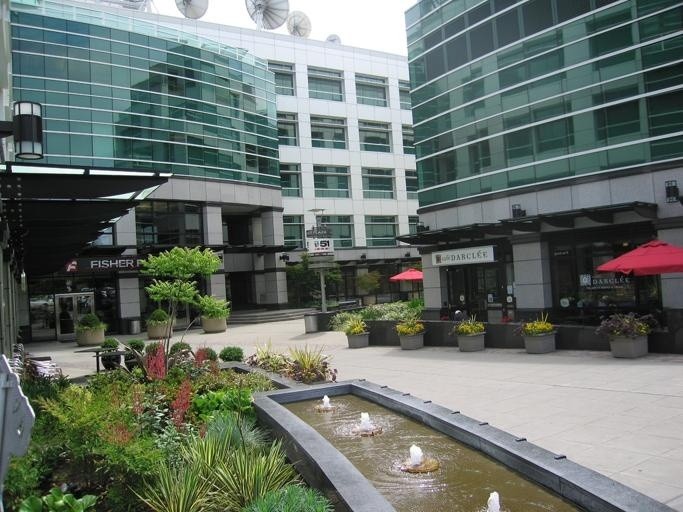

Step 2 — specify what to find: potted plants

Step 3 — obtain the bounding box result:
[74,313,105,346]
[394,313,424,350]
[594,313,651,358]
[101,338,144,371]
[343,317,370,348]
[146,309,173,338]
[455,317,486,352]
[519,319,556,354]
[200,302,227,333]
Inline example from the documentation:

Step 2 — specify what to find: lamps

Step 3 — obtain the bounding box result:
[510,204,525,219]
[415,222,428,234]
[0,99,43,162]
[279,253,289,263]
[663,180,680,204]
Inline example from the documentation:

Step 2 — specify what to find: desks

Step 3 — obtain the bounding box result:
[76,348,138,376]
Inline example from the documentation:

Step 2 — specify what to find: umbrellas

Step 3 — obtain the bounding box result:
[389,268,423,300]
[596,238,683,329]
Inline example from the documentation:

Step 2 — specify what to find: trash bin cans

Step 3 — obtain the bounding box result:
[304,312,319,334]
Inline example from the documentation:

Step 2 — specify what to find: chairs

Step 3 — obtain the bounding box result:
[11,342,64,386]
